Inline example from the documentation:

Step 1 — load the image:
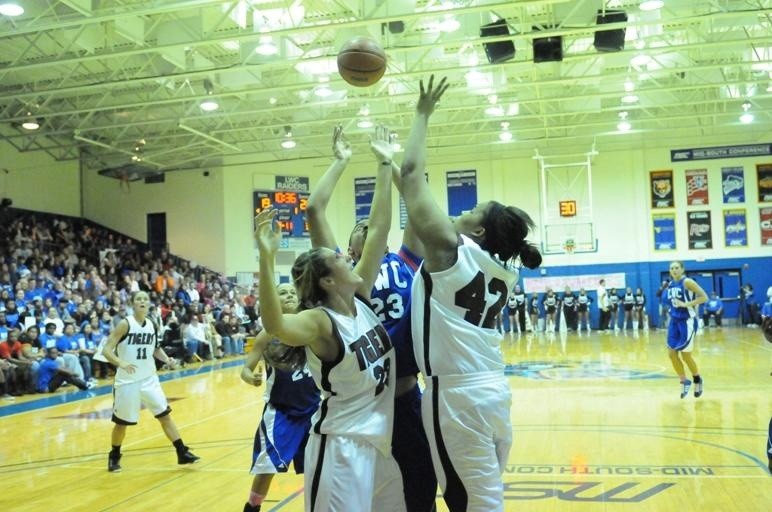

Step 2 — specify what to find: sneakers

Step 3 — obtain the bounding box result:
[693,376,703,397]
[680,378,691,399]
[751,324,758,331]
[89,377,99,384]
[12,392,23,396]
[23,385,35,393]
[90,376,98,384]
[86,381,95,390]
[177,448,204,464]
[746,323,753,327]
[108,449,122,472]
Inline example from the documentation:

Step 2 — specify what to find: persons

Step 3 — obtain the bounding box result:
[153,225,257,370]
[102,290,201,473]
[241,283,319,511]
[254,121,406,511]
[620,288,636,332]
[703,290,723,329]
[398,74,543,512]
[741,282,761,324]
[760,285,772,472]
[561,288,577,331]
[632,289,649,330]
[306,121,438,512]
[658,261,709,400]
[507,293,521,335]
[597,280,609,334]
[530,292,541,330]
[104,236,153,323]
[542,290,560,331]
[514,285,526,331]
[608,288,622,332]
[1,212,106,392]
[572,288,594,331]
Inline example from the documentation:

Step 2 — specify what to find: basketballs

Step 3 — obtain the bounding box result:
[337,36,388,88]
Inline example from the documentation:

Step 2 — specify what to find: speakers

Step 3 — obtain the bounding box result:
[532,24,563,63]
[593,9,628,52]
[480,19,516,65]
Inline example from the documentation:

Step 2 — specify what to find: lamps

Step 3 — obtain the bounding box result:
[198,96,220,112]
[19,113,41,131]
